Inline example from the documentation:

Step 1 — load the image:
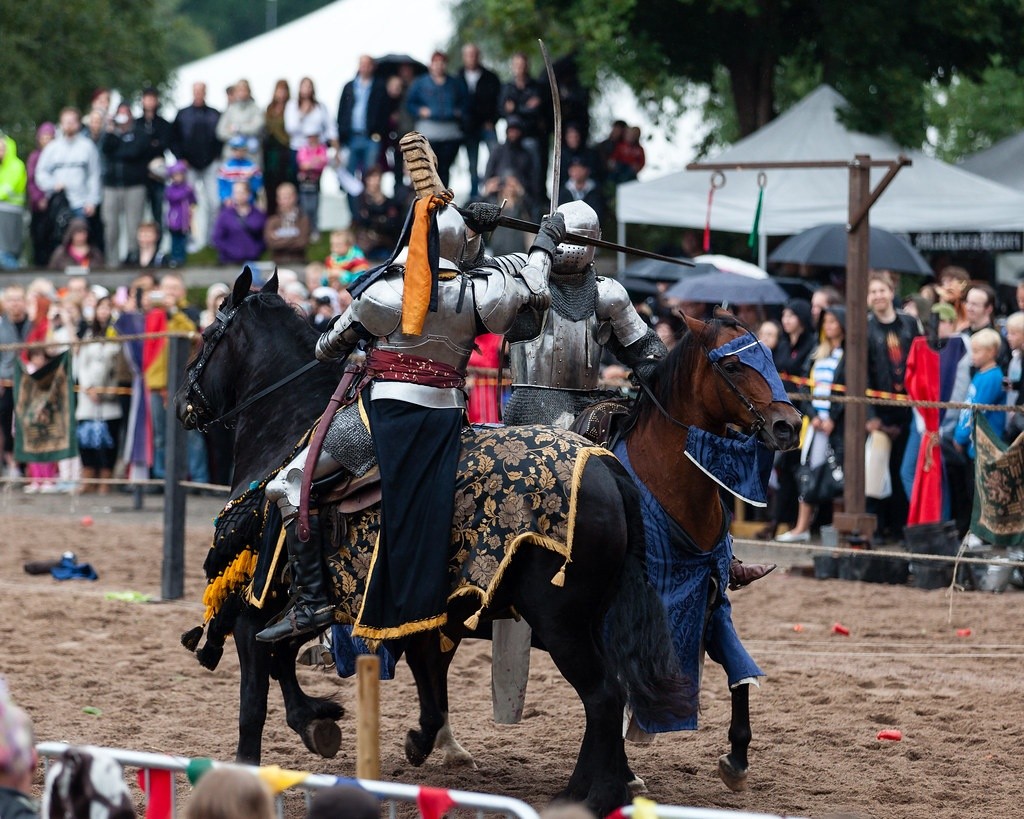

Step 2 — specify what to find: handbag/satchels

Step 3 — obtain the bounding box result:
[796,427,845,505]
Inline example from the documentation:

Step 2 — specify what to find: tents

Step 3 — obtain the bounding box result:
[157,0,655,141]
[615,81,1024,262]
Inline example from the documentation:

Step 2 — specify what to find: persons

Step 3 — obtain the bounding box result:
[0,229,370,495]
[251,197,566,645]
[456,198,777,591]
[0,680,42,819]
[1,41,646,273]
[178,765,381,819]
[751,264,1024,544]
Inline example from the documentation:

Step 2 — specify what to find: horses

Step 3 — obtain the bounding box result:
[177,260,700,819]
[509,308,802,792]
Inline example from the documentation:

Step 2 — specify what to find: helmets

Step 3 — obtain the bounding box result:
[436,206,472,266]
[549,198,600,273]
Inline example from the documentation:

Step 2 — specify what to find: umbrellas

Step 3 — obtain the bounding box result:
[762,221,933,275]
[623,251,787,306]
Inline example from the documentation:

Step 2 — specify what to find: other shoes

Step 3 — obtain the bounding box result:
[728,558,778,591]
[777,528,813,543]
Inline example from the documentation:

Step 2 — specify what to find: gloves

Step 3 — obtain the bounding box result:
[525,211,566,262]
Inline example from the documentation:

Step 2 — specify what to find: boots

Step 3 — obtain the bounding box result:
[255,505,336,643]
